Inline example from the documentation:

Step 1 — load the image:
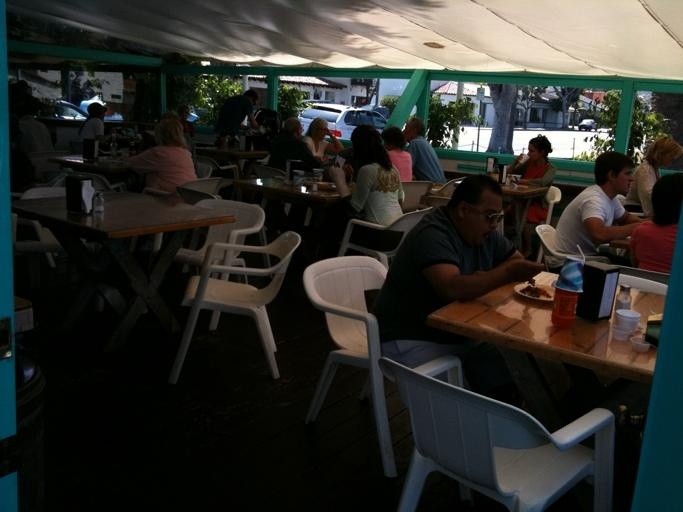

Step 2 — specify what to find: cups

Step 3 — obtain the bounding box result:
[293,170,305,184]
[517,153,529,164]
[313,169,324,182]
[551,262,584,327]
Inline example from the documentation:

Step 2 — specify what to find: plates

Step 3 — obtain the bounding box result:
[514,283,554,300]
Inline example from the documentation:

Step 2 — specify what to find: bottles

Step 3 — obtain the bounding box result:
[614,282,634,314]
[91,189,104,215]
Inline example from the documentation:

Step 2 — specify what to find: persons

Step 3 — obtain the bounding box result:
[627,135,683,214]
[371,173,543,409]
[79,103,106,147]
[10,78,60,188]
[402,118,447,187]
[132,117,197,196]
[329,126,406,253]
[631,172,683,274]
[544,151,646,270]
[220,89,258,146]
[258,106,283,135]
[303,116,342,164]
[506,136,555,257]
[270,119,327,173]
[382,125,413,184]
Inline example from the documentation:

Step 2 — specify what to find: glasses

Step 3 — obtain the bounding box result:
[464,205,504,223]
[316,128,328,131]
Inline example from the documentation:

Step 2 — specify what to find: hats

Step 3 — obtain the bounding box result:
[87,102,106,114]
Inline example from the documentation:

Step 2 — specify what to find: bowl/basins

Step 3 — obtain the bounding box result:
[509,175,521,184]
[630,336,650,352]
[613,328,633,341]
[615,309,642,330]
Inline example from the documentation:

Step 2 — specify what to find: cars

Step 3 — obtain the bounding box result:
[298,103,387,140]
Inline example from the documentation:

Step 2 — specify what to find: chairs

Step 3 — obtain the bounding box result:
[25,149,72,183]
[377,356,616,512]
[398,180,434,213]
[166,231,301,385]
[422,176,467,206]
[536,222,611,264]
[338,204,434,265]
[154,199,266,330]
[130,177,222,253]
[615,265,670,287]
[18,187,67,269]
[536,185,562,263]
[60,171,127,193]
[256,165,289,178]
[176,187,223,205]
[301,256,463,478]
[192,155,240,195]
[614,194,645,218]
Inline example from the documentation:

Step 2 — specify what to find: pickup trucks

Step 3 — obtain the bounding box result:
[578,119,598,132]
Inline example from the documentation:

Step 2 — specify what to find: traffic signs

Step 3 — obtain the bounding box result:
[476,88,485,101]
